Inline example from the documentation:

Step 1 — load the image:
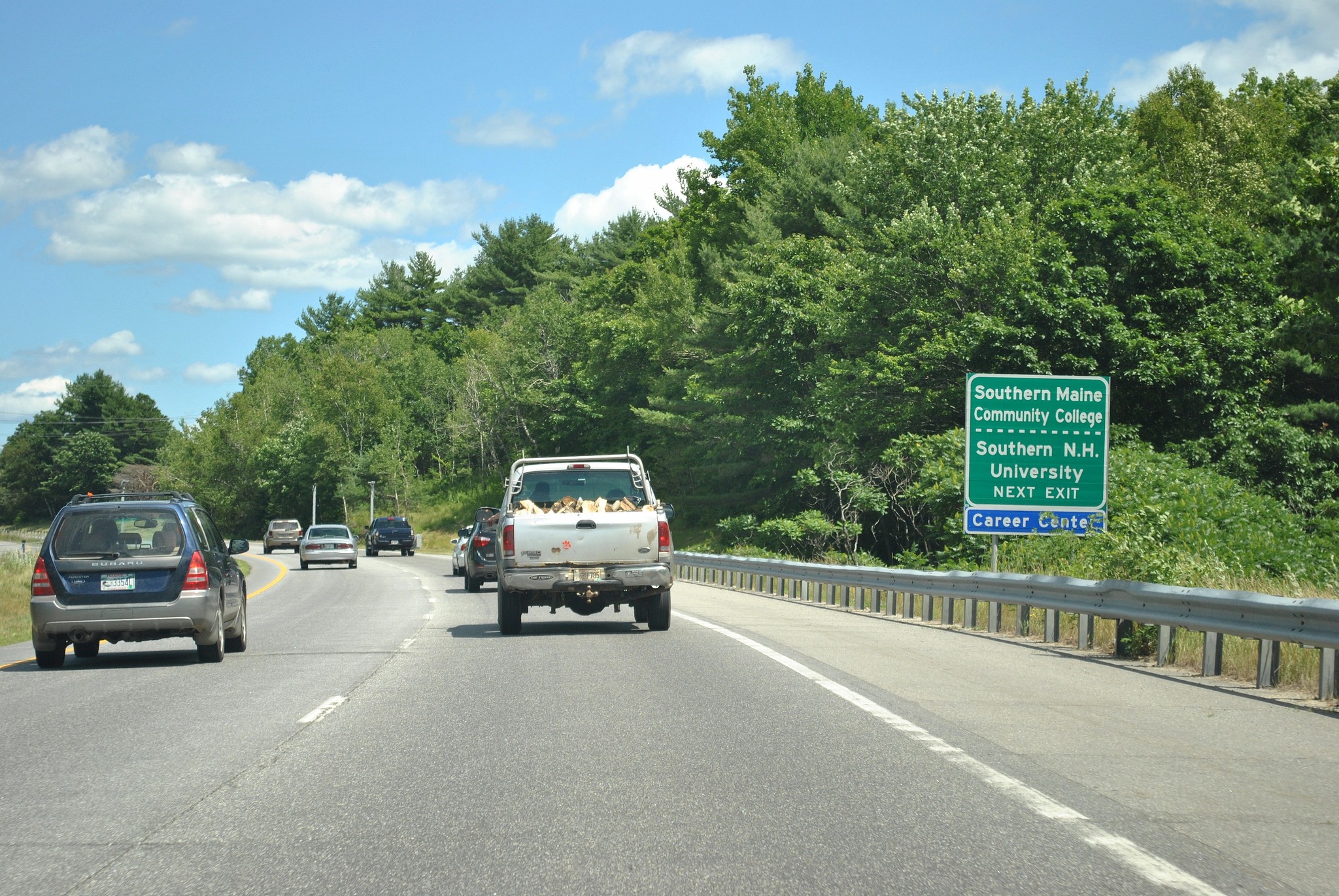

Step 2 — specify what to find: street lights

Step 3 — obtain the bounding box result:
[121,479,129,533]
[368,481,375,525]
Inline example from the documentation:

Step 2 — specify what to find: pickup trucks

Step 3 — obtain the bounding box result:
[364,517,415,557]
[475,445,676,635]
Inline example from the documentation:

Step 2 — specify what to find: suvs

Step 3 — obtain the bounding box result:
[458,508,501,592]
[261,518,303,554]
[29,491,250,669]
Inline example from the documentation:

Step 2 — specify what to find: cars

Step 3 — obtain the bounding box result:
[451,524,473,577]
[295,524,359,570]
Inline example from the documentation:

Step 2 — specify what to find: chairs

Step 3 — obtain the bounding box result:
[606,489,625,504]
[530,482,551,502]
[79,511,181,557]
